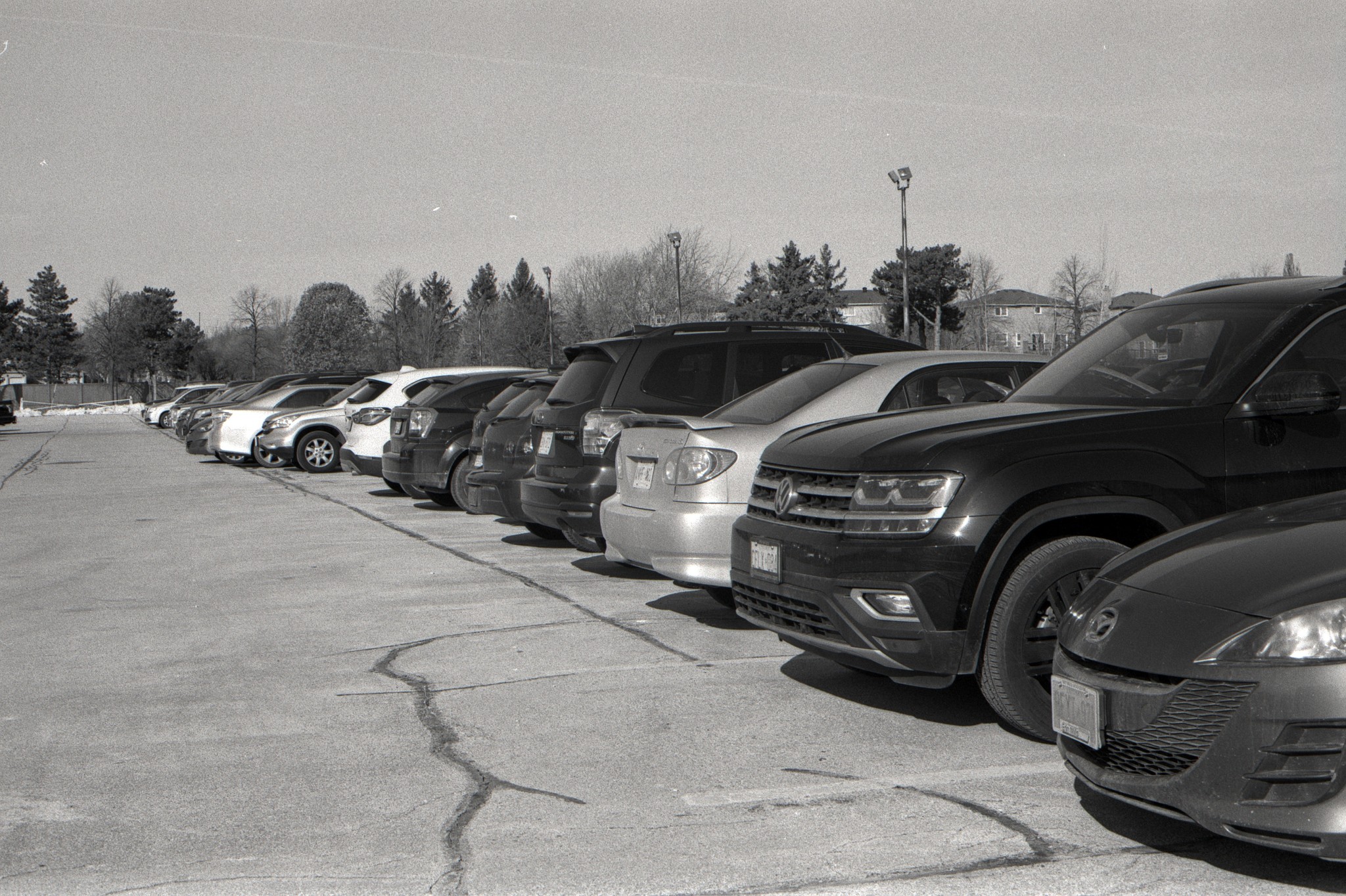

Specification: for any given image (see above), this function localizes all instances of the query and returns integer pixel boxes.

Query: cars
[143,273,1346,866]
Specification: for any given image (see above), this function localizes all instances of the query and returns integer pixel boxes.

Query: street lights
[542,267,554,364]
[666,233,682,322]
[889,168,914,342]
